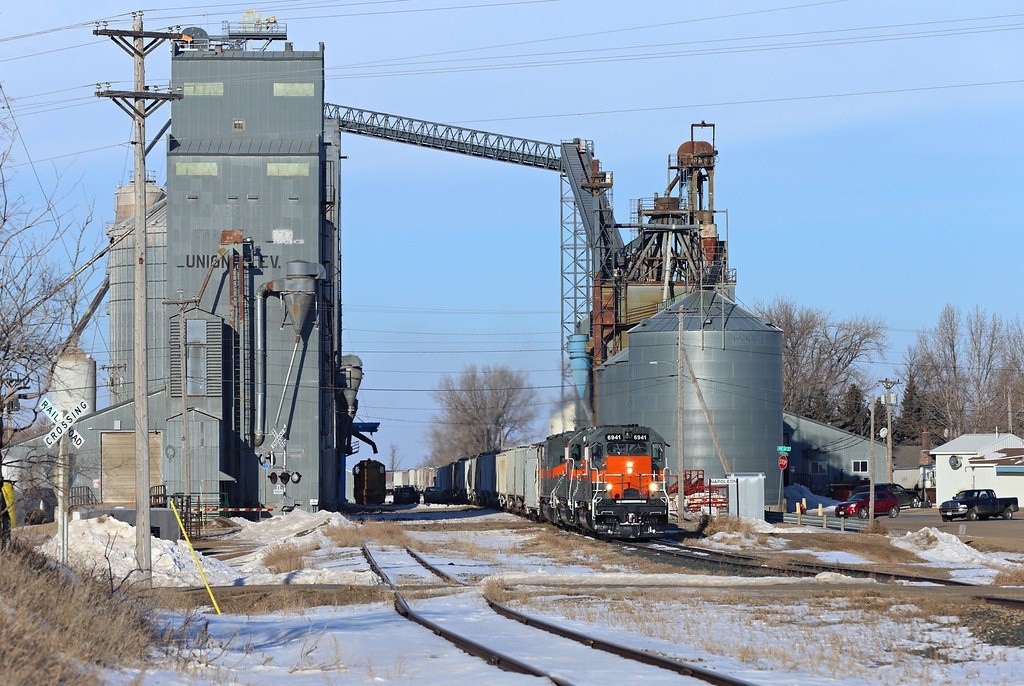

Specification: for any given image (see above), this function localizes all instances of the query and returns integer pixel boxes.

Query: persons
[914,481,918,491]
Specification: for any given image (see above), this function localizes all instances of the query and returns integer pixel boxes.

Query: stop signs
[777,456,788,470]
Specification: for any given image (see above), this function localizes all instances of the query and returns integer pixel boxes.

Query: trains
[386,425,669,541]
[353,459,387,505]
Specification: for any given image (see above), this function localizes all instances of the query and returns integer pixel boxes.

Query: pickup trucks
[938,489,1018,521]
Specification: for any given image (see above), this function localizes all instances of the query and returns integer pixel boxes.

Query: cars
[423,485,451,504]
[393,485,420,505]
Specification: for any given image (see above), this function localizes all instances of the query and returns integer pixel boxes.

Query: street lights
[649,360,684,529]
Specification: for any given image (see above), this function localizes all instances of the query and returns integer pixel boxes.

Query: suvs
[853,484,921,509]
[835,491,901,520]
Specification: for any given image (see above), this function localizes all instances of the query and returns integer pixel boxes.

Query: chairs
[981,494,986,497]
